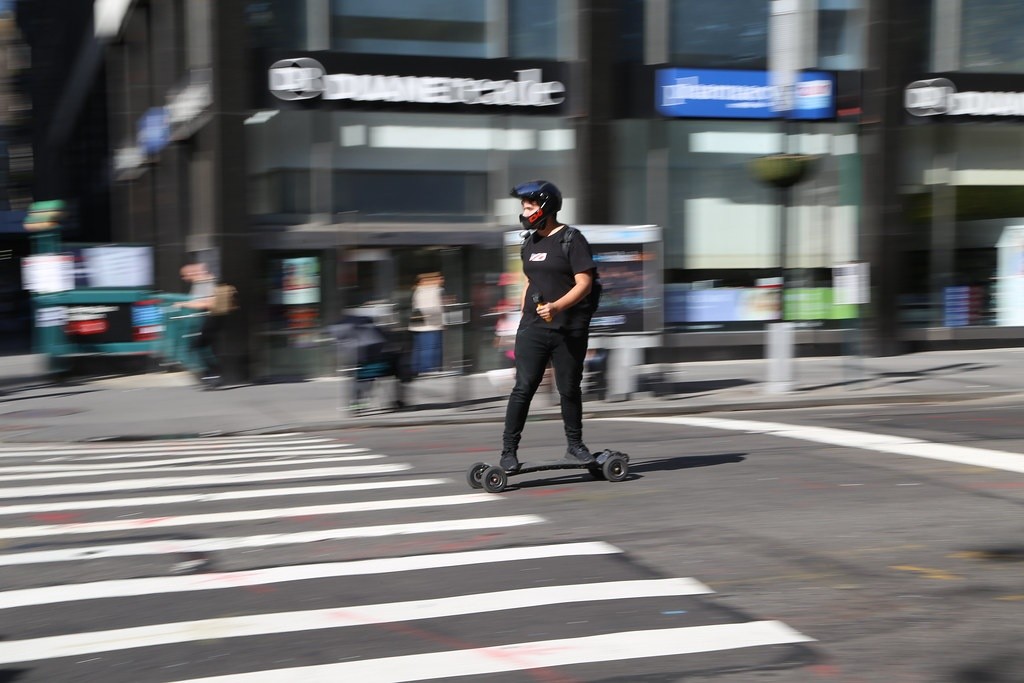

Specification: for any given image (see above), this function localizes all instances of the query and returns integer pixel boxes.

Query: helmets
[509,179,562,230]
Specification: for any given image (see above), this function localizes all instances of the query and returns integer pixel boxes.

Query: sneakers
[499,452,520,471]
[564,444,594,464]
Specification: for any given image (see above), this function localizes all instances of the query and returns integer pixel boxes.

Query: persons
[498,180,603,470]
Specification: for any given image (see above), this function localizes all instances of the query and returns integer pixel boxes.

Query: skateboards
[466,448,630,492]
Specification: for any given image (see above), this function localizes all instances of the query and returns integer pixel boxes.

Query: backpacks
[565,230,603,311]
